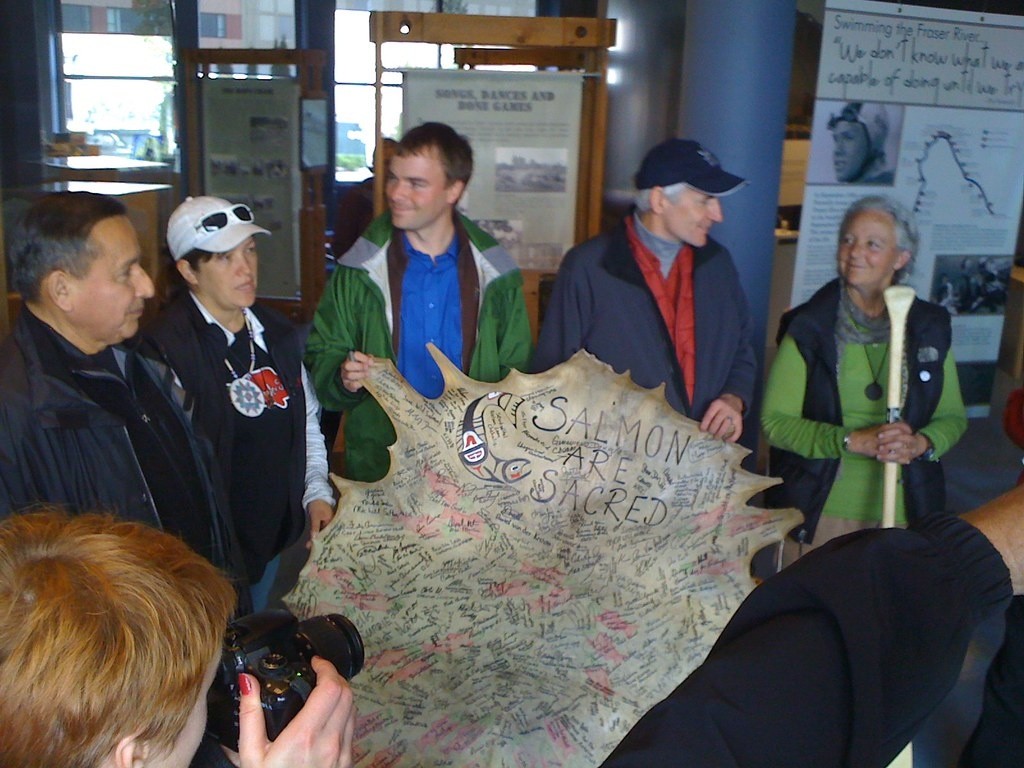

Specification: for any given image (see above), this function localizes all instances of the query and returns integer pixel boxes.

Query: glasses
[827,108,871,146]
[178,203,253,257]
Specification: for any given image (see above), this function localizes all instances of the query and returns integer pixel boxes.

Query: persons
[588,487,1024,768]
[956,388,1024,768]
[0,513,356,768]
[532,136,756,445]
[0,190,253,615]
[332,139,399,262]
[305,122,533,482]
[142,195,337,612]
[826,101,896,186]
[760,193,967,565]
[937,257,1005,316]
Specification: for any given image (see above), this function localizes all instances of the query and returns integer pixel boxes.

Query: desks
[1,180,173,314]
[41,155,171,181]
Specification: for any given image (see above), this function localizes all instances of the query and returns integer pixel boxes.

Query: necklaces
[848,310,889,401]
[225,308,274,417]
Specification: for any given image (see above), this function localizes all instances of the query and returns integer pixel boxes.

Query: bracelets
[842,433,850,448]
[916,445,933,461]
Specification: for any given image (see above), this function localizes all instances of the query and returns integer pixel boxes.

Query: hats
[167,196,272,260]
[633,138,750,197]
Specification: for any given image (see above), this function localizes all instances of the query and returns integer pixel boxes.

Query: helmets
[828,100,890,162]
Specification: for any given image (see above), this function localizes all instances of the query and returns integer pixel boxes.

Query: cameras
[202,604,365,754]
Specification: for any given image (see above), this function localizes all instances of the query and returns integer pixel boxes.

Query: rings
[889,449,896,454]
[728,417,732,421]
